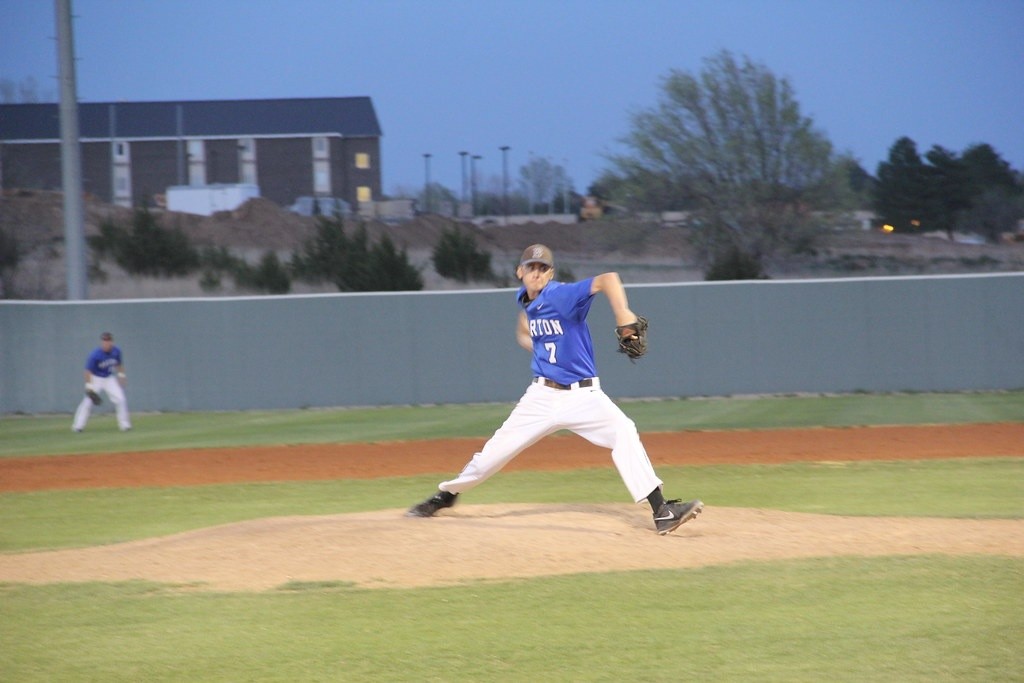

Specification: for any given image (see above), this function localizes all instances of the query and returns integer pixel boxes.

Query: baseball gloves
[86,389,103,406]
[615,316,648,360]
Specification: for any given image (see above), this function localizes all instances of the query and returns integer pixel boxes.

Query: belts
[534,376,592,390]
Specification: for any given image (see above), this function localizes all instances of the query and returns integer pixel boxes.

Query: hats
[100,332,113,341]
[519,244,553,267]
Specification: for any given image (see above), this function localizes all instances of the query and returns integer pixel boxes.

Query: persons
[71,332,132,434]
[408,243,706,538]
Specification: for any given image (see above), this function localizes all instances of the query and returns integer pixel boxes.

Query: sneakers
[653,497,704,535]
[406,492,458,518]
